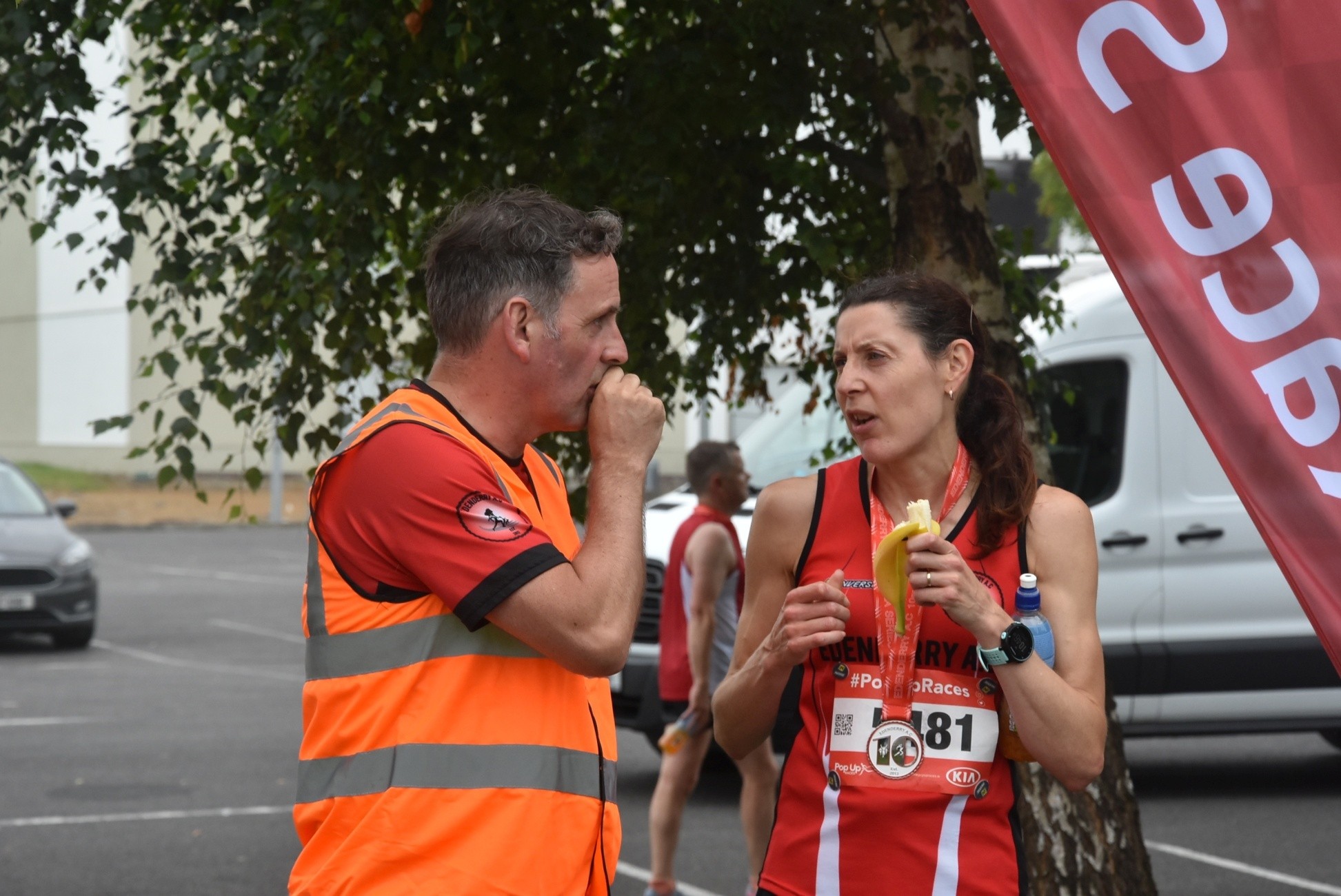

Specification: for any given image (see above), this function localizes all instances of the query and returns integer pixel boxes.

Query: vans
[608,247,1341,779]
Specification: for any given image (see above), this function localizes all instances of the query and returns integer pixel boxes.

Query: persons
[286,185,666,896]
[710,272,1108,896]
[642,440,779,896]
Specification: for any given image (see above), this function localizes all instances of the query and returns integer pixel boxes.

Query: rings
[926,571,933,588]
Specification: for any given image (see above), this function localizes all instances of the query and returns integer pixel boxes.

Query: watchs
[975,621,1035,672]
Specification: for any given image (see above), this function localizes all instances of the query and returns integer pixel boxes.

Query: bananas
[873,499,941,635]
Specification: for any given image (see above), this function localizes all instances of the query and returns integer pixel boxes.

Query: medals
[867,719,924,780]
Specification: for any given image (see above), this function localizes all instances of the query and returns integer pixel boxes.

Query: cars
[0,461,100,653]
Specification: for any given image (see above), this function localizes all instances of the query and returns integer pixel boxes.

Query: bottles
[996,573,1056,763]
[657,710,696,757]
